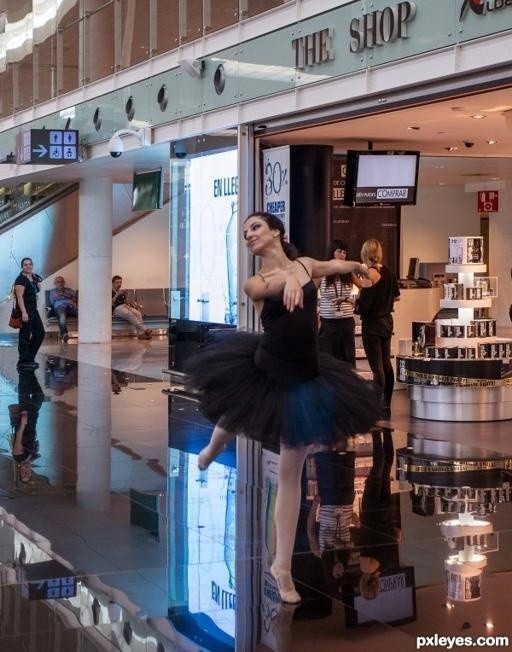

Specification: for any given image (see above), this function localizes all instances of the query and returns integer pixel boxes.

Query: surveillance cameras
[107,124,152,158]
[173,141,187,159]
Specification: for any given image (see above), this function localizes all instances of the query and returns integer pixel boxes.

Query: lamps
[178,60,205,77]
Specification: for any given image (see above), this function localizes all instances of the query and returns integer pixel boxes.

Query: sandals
[198,442,226,471]
[269,563,301,603]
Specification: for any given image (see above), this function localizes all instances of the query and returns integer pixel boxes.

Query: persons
[12,368,45,484]
[49,275,78,342]
[306,421,400,600]
[12,257,45,368]
[313,238,401,420]
[112,275,152,340]
[49,358,74,396]
[111,340,153,395]
[197,211,370,604]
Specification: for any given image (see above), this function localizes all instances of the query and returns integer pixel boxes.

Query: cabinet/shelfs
[396,433,511,568]
[396,264,512,421]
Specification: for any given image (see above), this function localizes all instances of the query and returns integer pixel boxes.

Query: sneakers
[16,366,39,375]
[383,407,391,418]
[16,360,40,367]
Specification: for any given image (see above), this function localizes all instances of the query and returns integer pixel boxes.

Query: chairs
[44,288,169,338]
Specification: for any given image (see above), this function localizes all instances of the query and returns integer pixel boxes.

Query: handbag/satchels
[8,298,23,329]
[8,404,21,434]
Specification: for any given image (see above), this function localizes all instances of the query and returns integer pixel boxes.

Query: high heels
[271,603,301,636]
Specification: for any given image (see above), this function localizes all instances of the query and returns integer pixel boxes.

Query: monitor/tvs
[130,487,161,538]
[408,257,421,281]
[343,150,420,207]
[344,565,417,631]
[132,166,164,211]
